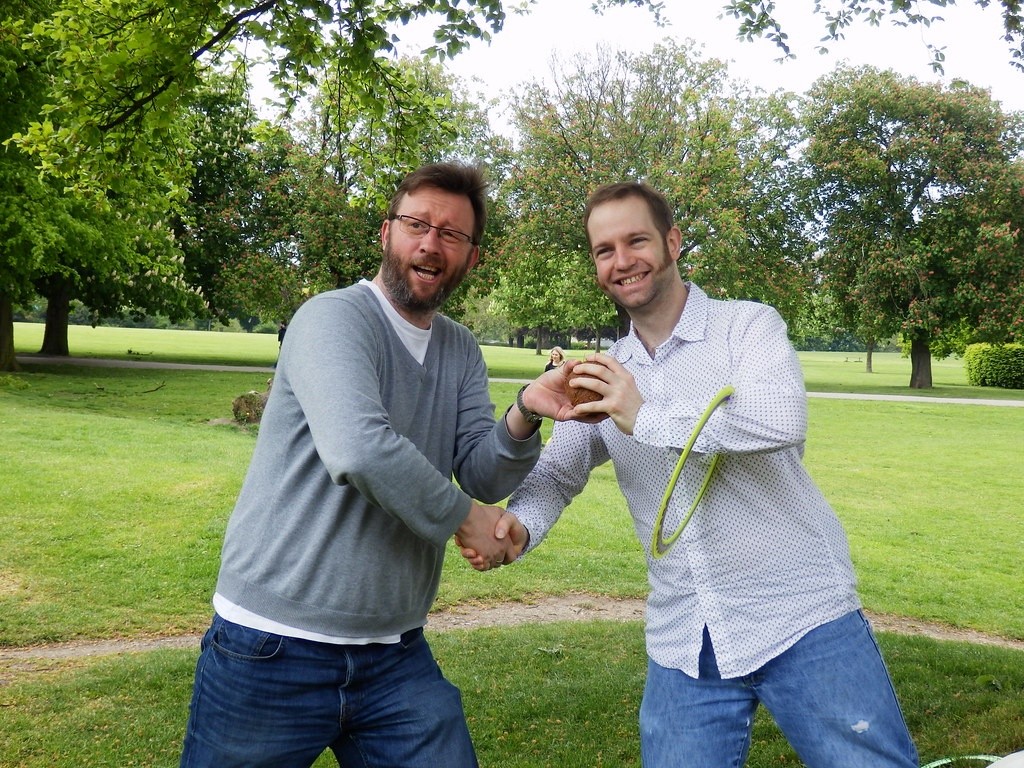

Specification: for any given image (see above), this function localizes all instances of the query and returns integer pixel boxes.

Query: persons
[180,164,588,767]
[455,180,919,767]
[545,346,565,372]
[274,320,288,367]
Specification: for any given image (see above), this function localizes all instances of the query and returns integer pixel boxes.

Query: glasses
[388,215,478,252]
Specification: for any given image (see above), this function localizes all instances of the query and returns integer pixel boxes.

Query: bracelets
[516,384,541,424]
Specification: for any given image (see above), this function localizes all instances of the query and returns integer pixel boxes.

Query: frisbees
[651,383,736,561]
